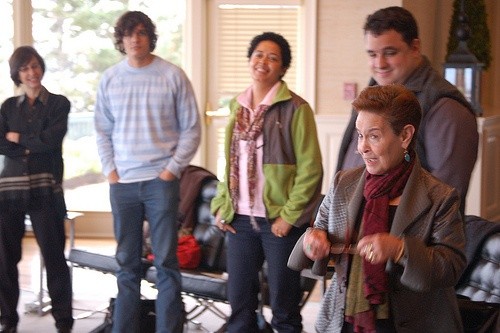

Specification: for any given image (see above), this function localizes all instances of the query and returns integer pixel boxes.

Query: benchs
[451,217,500,333]
[67,173,323,333]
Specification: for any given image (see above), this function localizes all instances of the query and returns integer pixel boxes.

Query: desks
[24,210,86,315]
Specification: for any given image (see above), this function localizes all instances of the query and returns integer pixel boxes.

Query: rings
[305,243,311,252]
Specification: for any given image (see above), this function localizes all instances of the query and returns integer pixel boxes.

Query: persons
[0,45,74,333]
[335,6,479,216]
[93,11,202,333]
[210,31,324,333]
[286,84,470,333]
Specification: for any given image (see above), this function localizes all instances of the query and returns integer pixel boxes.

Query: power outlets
[343,82,357,101]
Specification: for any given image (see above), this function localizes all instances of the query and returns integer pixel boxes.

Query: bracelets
[393,241,405,263]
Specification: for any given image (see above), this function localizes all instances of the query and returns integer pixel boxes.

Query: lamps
[439,0,491,69]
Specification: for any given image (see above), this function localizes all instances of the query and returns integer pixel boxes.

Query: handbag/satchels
[104,297,187,333]
[148,236,202,268]
